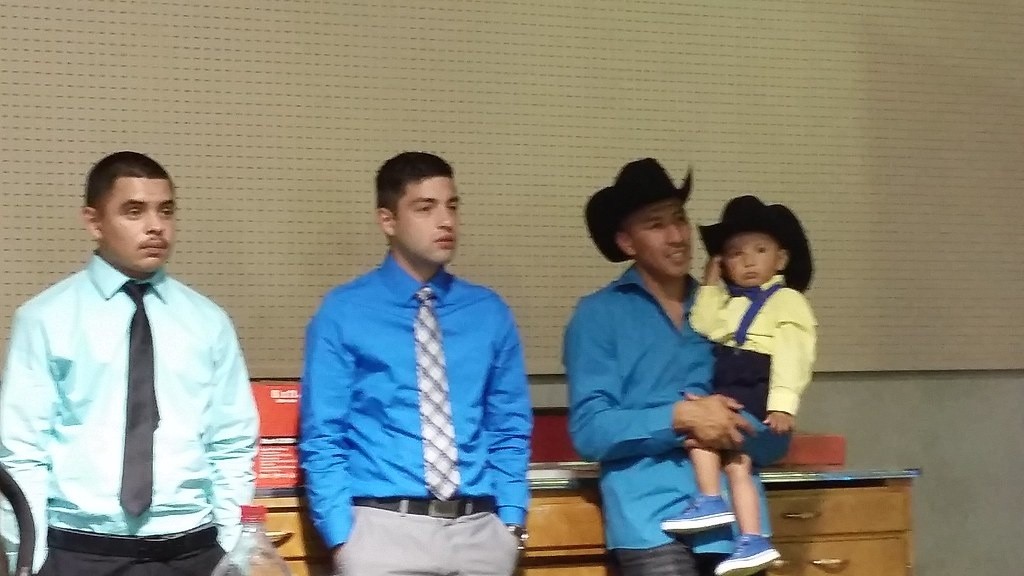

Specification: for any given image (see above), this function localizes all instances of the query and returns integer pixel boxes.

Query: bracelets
[507,525,531,542]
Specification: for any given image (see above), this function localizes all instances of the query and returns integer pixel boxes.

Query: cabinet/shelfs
[230,464,929,576]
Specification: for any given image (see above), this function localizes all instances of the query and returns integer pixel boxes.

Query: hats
[586,158,693,263]
[699,195,811,294]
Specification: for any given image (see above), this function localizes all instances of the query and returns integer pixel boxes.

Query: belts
[49,526,218,560]
[351,496,496,518]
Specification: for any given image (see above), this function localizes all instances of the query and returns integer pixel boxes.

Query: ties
[120,283,160,518]
[414,287,462,502]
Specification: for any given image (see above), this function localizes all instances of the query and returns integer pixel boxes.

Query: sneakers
[714,534,781,576]
[661,493,736,534]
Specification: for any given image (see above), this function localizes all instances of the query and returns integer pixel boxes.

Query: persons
[660,194,819,576]
[297,151,534,576]
[0,150,262,576]
[563,156,794,576]
